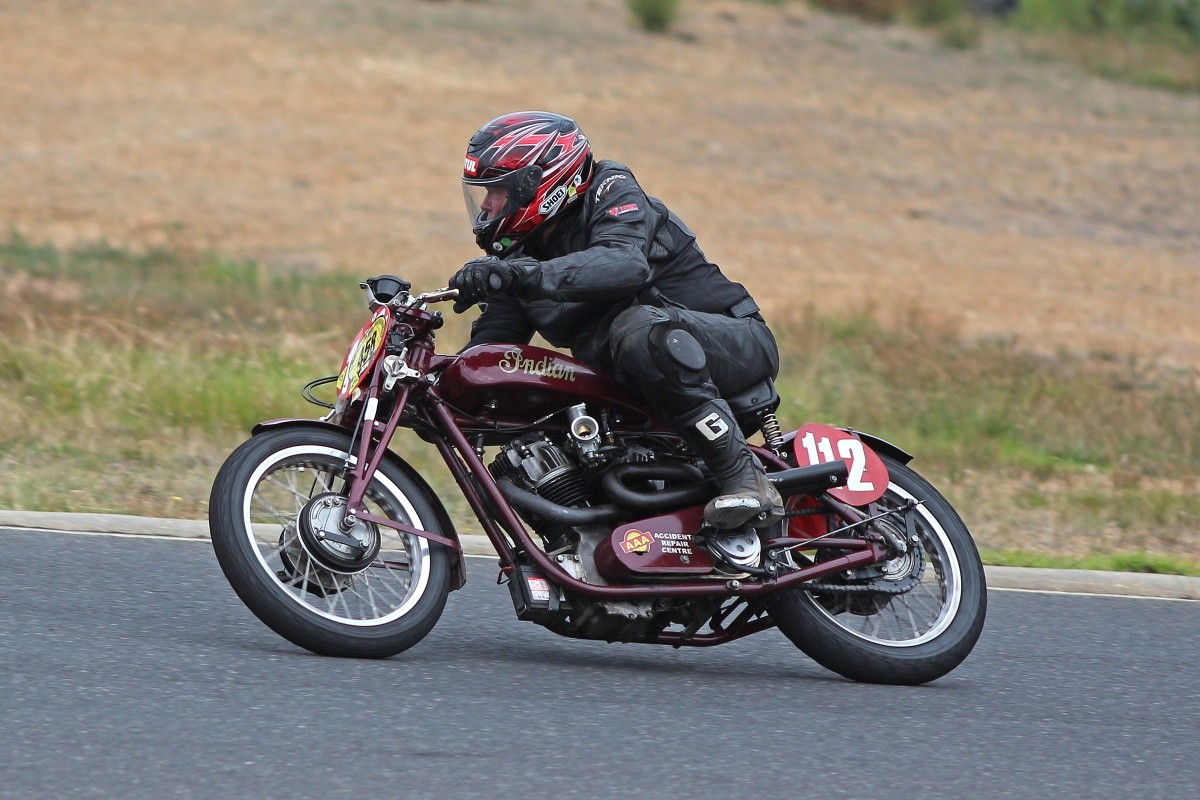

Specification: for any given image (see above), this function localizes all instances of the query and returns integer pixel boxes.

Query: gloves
[448,248,543,314]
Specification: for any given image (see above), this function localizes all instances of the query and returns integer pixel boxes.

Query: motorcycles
[208,272,987,686]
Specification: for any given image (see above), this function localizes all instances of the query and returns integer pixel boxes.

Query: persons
[449,111,786,534]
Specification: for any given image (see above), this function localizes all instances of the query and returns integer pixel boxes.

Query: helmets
[460,111,596,258]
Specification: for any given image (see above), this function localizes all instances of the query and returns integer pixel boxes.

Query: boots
[671,397,785,528]
